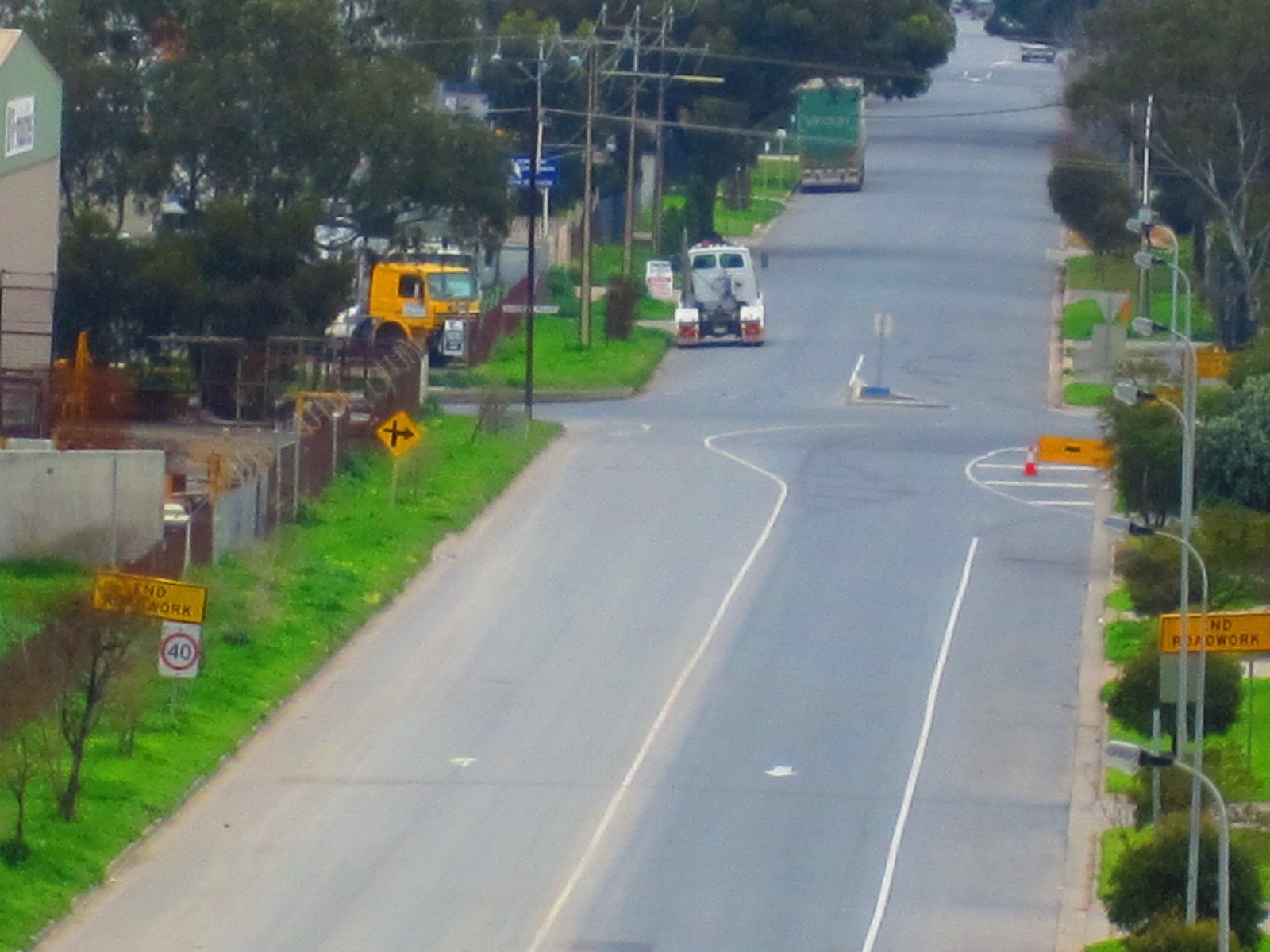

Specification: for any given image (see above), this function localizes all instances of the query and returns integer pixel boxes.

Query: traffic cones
[1024,447,1037,477]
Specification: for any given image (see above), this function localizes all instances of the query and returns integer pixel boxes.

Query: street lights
[490,54,580,426]
[1101,515,1209,931]
[1110,378,1194,764]
[1100,738,1230,952]
[1110,216,1198,526]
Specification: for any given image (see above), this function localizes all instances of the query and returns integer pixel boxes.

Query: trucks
[797,75,865,194]
[324,237,483,367]
[674,239,769,350]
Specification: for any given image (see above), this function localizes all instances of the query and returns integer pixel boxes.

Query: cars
[1020,41,1056,63]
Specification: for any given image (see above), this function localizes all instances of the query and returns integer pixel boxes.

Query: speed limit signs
[158,621,202,680]
